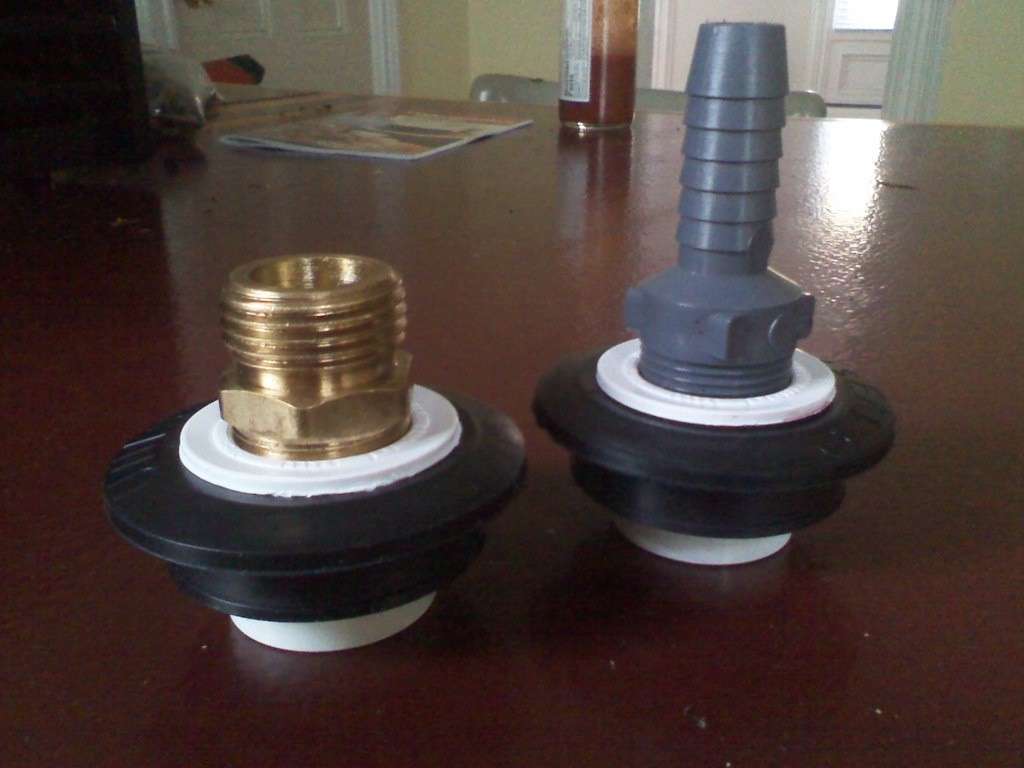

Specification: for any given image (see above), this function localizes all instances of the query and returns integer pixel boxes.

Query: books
[220,108,534,160]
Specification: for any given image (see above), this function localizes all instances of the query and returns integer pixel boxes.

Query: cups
[559,0,639,129]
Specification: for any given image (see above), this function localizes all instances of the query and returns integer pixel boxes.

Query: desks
[0,80,1024,768]
